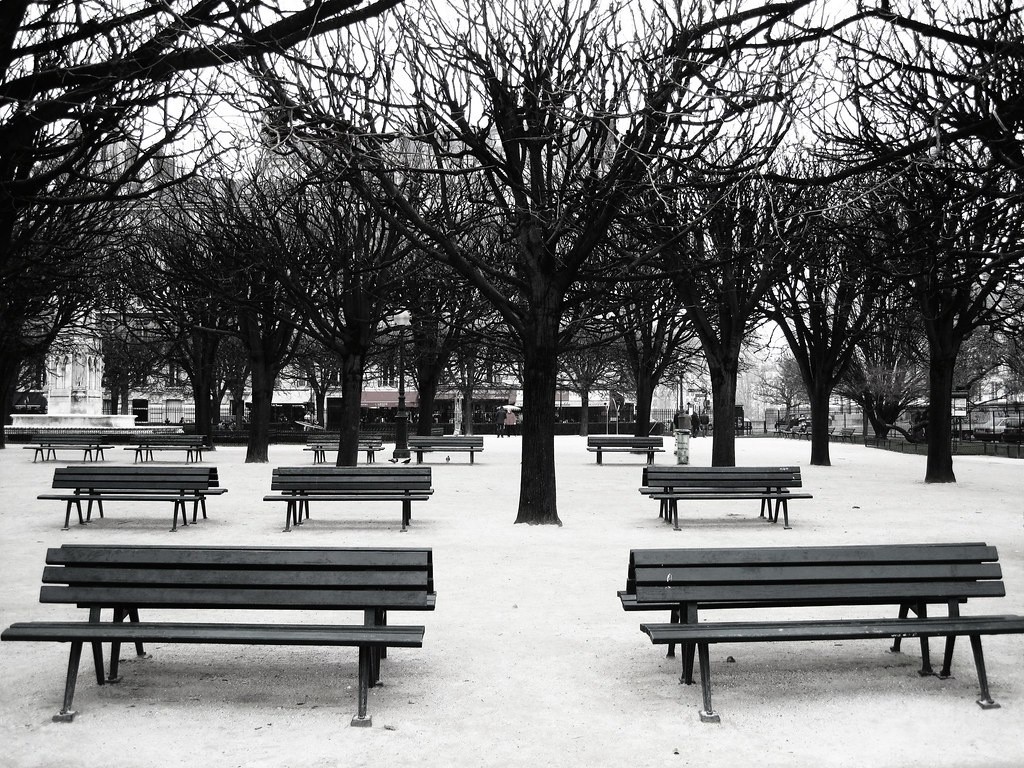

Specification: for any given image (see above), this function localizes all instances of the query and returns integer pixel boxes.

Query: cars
[974,417,1024,441]
[774,413,833,430]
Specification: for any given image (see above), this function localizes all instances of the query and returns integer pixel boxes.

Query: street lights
[390,308,412,464]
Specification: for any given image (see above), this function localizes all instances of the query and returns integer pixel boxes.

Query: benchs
[639,465,813,531]
[37,466,228,532]
[124,435,213,465]
[1,545,437,728]
[405,436,483,465]
[772,425,856,445]
[23,434,114,464]
[304,434,386,465]
[263,466,434,532]
[586,437,665,466]
[617,542,1024,723]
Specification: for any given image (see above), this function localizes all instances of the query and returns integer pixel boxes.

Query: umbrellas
[496,405,521,412]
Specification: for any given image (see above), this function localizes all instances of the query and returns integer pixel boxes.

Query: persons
[278,414,288,424]
[504,409,517,437]
[684,410,692,429]
[673,410,680,434]
[241,417,250,423]
[691,412,699,438]
[699,410,709,437]
[216,418,236,431]
[179,417,185,424]
[164,418,170,426]
[494,407,507,438]
